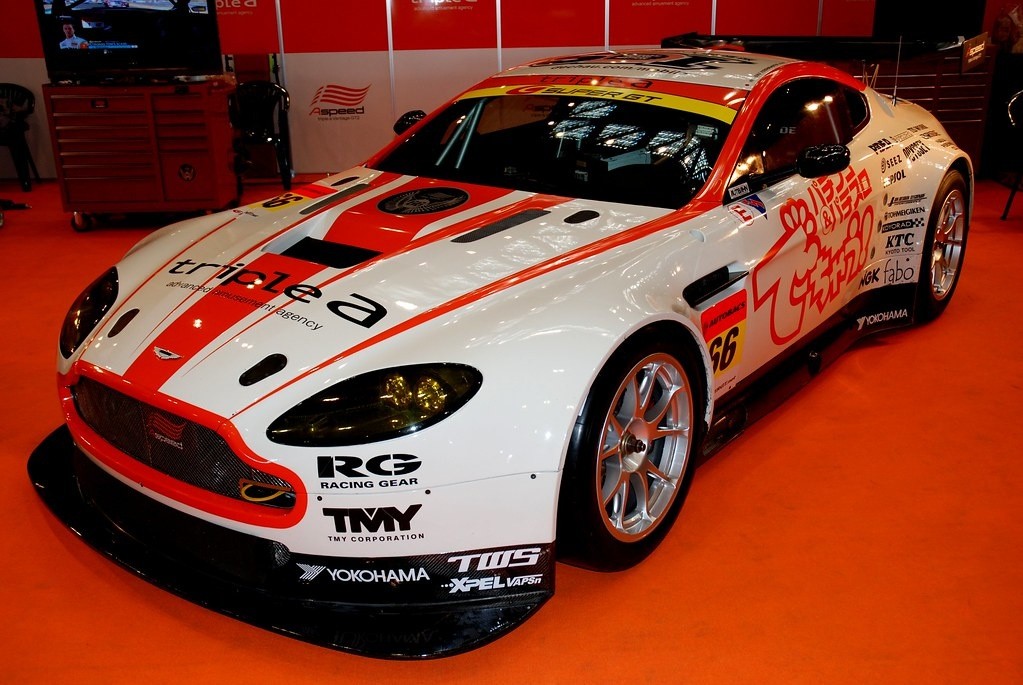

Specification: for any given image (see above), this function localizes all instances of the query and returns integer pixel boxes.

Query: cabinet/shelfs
[853,32,1000,176]
[42,78,241,232]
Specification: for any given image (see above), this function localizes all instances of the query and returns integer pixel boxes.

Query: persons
[59,23,88,48]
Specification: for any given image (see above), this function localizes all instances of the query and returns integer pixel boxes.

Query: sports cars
[27,48,974,661]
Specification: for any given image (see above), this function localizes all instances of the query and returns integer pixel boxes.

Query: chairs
[0,82,41,192]
[228,79,292,196]
[1001,89,1023,221]
